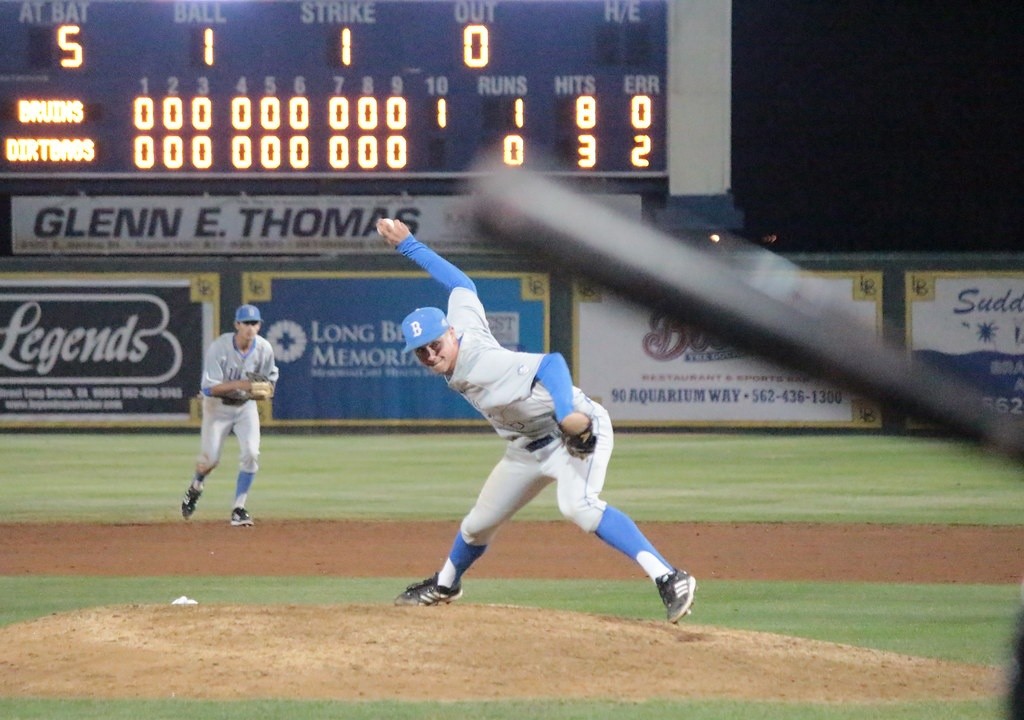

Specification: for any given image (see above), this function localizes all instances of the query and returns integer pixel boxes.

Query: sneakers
[231,507,253,526]
[394,573,463,607]
[656,567,697,624]
[181,481,203,518]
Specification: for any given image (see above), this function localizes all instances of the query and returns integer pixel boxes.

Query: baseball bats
[459,134,1024,464]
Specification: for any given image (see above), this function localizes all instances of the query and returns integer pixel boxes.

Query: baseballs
[376,217,396,237]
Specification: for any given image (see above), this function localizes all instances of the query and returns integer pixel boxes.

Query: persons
[375,216,697,624]
[182,305,278,528]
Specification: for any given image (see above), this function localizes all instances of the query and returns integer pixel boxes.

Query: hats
[401,307,450,355]
[236,304,260,322]
